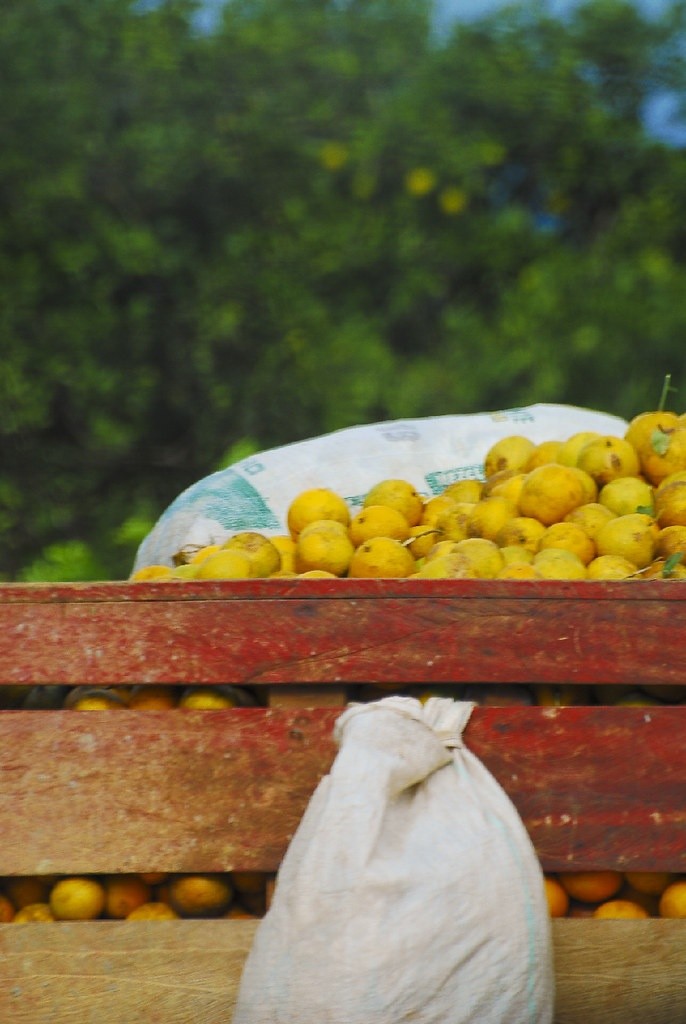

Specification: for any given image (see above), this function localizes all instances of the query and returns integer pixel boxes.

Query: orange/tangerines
[0,410,686,924]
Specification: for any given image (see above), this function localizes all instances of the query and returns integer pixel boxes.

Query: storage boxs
[0,577,686,1024]
